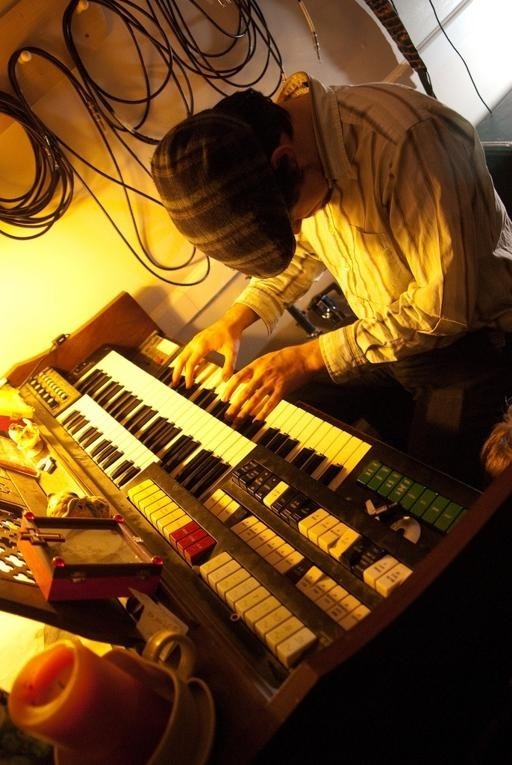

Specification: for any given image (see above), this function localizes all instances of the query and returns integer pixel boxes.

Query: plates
[177,676,217,764]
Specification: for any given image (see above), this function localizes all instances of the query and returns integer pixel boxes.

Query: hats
[149,107,298,280]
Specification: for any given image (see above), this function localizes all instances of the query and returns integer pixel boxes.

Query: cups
[55,631,196,764]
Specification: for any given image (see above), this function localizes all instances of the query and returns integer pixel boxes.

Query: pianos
[4,287,509,741]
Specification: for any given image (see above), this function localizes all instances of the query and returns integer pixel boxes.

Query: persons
[142,70,512,425]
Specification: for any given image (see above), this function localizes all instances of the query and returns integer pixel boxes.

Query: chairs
[475,141,512,217]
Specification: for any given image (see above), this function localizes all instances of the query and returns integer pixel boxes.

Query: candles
[7,633,167,759]
